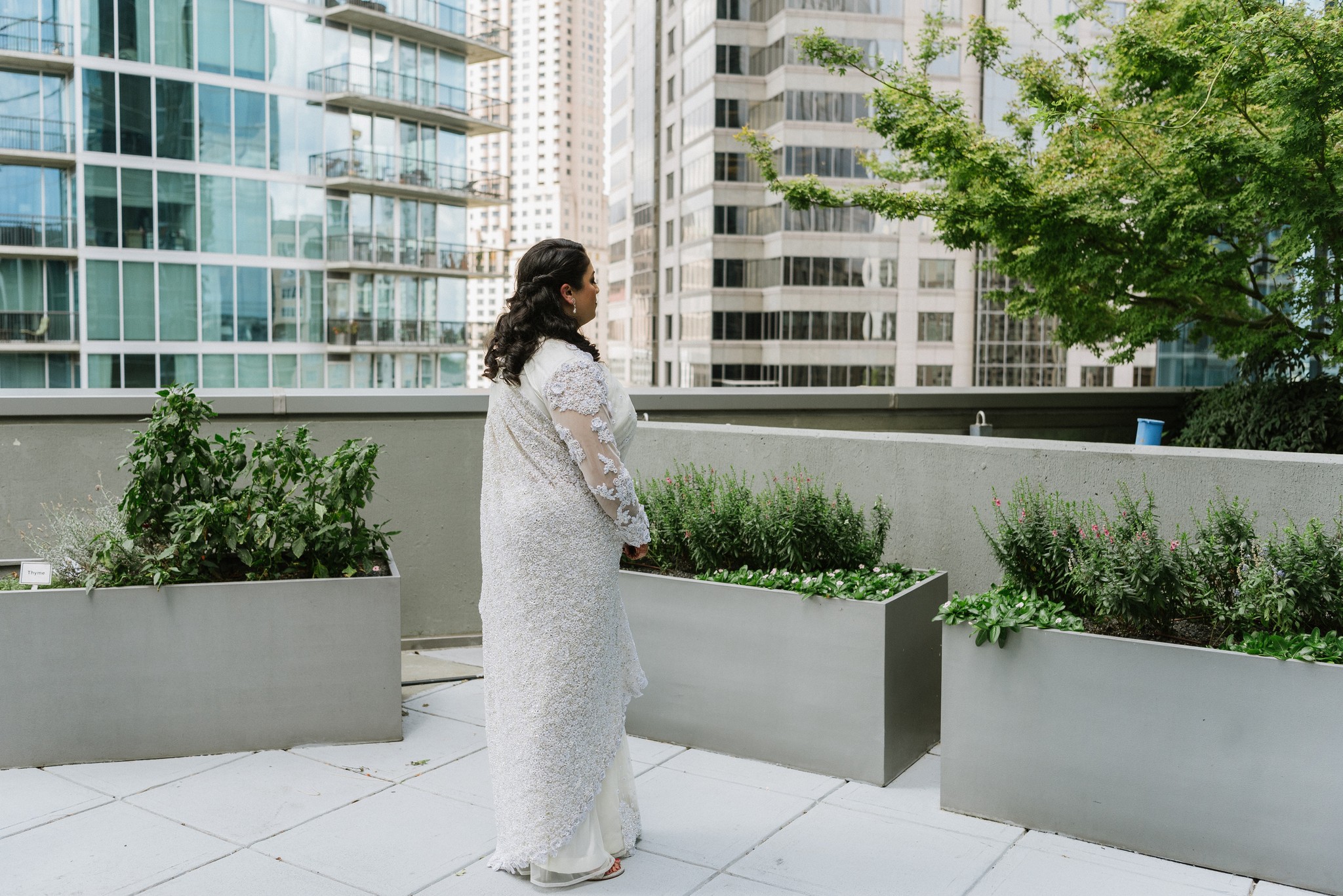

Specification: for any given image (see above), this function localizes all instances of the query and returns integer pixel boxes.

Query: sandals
[577,857,625,881]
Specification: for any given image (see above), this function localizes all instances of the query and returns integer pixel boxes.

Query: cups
[1134,417,1164,446]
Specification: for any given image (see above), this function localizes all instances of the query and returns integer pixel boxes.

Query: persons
[478,238,651,882]
